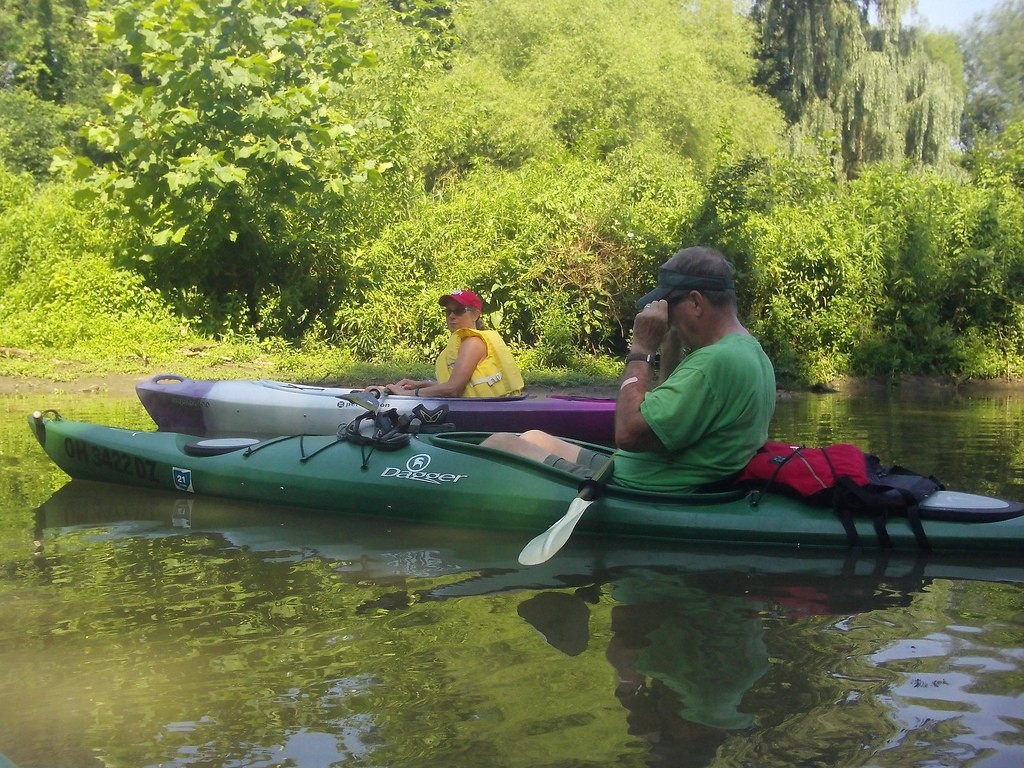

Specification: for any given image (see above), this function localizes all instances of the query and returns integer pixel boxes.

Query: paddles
[517,452,614,568]
[334,387,391,413]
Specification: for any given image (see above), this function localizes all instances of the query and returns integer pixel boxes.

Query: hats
[438,289,482,312]
[636,265,729,308]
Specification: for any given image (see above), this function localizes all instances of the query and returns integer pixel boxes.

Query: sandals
[385,403,456,434]
[345,410,411,452]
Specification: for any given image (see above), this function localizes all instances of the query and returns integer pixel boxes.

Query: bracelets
[624,353,655,368]
[415,388,420,397]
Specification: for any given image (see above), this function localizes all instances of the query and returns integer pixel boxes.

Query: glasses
[442,308,478,317]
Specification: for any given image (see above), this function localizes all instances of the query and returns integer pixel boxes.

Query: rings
[644,305,651,308]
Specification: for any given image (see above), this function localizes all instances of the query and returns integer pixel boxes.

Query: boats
[29,470,1024,594]
[25,407,1024,553]
[134,373,617,452]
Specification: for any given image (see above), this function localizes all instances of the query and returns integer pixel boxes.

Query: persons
[478,248,776,495]
[350,290,524,398]
